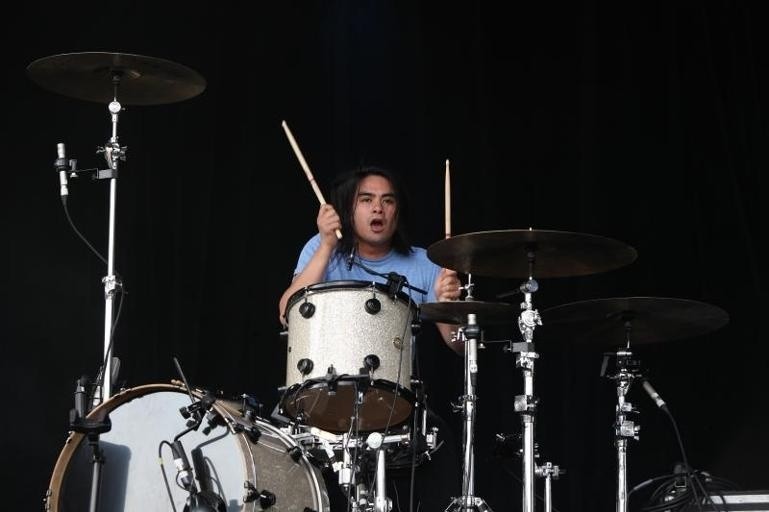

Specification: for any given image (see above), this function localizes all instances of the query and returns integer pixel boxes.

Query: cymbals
[543,297,729,347]
[418,302,517,326]
[427,230,640,280]
[29,52,205,107]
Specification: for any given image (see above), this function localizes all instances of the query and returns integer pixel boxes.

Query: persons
[279,166,466,512]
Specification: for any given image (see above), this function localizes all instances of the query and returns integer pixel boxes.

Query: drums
[46,384,332,510]
[282,280,415,433]
